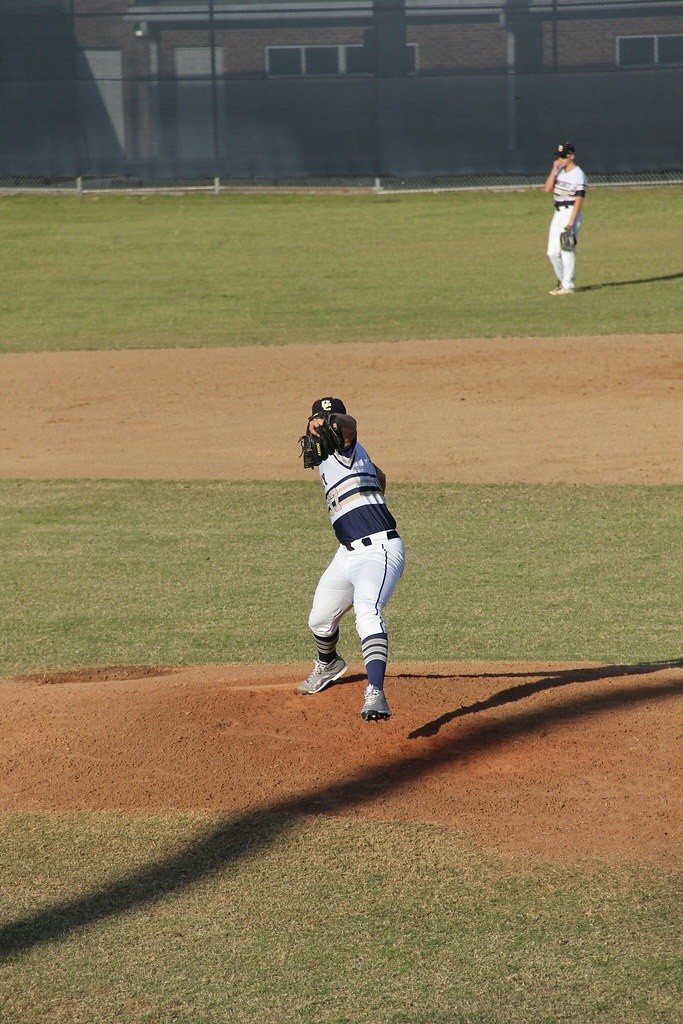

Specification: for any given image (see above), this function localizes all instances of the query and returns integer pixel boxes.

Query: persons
[543,142,588,296]
[296,398,405,722]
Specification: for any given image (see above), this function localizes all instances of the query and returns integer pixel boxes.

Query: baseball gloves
[559,224,578,252]
[296,411,358,471]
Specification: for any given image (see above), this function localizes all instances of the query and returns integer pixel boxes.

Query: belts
[555,205,568,210]
[346,531,399,551]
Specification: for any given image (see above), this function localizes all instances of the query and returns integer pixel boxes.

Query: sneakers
[549,282,575,296]
[360,683,391,723]
[295,656,347,694]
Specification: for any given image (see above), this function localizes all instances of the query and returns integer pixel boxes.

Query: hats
[555,142,574,158]
[308,397,346,418]
